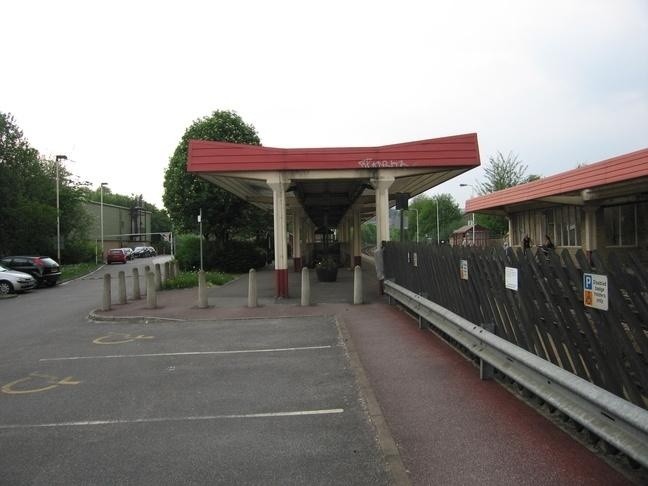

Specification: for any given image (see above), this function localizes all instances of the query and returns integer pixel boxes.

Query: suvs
[0,255,62,289]
[106,249,127,265]
[132,245,151,258]
[148,246,157,257]
[121,247,135,261]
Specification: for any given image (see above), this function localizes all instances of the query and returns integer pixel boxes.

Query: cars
[0,264,37,298]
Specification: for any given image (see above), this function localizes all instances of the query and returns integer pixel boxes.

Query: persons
[541,235,554,257]
[522,234,531,256]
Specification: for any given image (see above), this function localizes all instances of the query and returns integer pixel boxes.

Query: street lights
[459,183,475,246]
[426,197,440,247]
[55,154,69,269]
[100,182,108,263]
[408,207,420,244]
